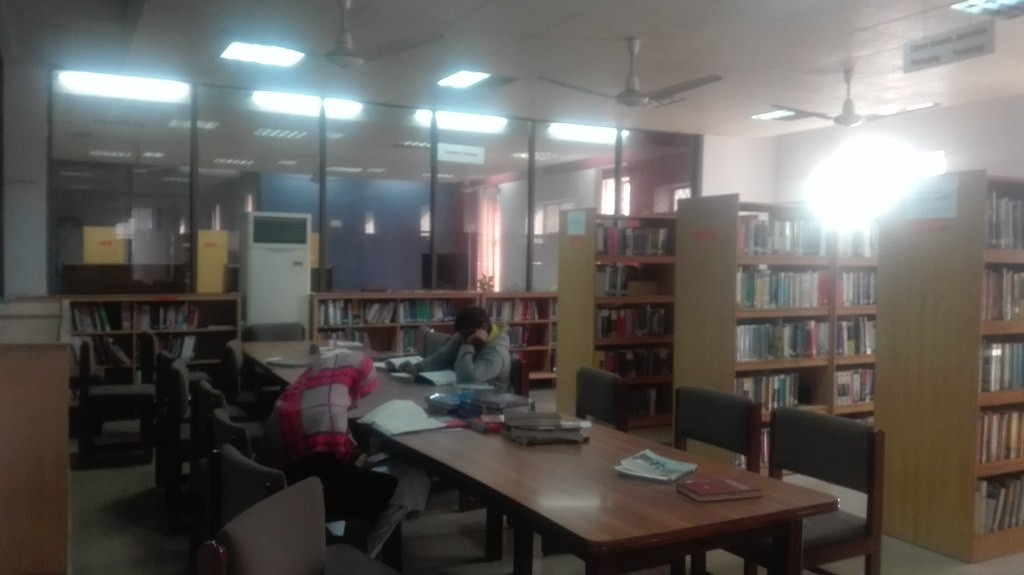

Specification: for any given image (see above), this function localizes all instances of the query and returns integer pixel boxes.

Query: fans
[772,68,942,127]
[272,0,446,85]
[537,37,724,108]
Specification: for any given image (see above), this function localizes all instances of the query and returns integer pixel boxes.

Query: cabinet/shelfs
[311,291,481,358]
[868,167,1024,564]
[674,192,877,477]
[558,207,675,430]
[481,292,557,391]
[57,291,242,437]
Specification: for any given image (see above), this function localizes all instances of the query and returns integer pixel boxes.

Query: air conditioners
[239,209,314,335]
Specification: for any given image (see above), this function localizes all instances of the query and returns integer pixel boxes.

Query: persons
[397,306,512,396]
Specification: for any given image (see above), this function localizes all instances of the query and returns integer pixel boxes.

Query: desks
[243,337,841,575]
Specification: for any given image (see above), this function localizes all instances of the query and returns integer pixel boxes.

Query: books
[73,299,200,365]
[317,297,558,373]
[737,216,878,471]
[676,477,764,503]
[423,391,536,435]
[594,223,673,380]
[977,191,1024,536]
[613,448,698,484]
[372,352,495,393]
[502,408,593,448]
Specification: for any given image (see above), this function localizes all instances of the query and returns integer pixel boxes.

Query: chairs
[222,338,280,422]
[458,356,530,514]
[506,366,629,558]
[190,378,229,564]
[220,444,399,575]
[242,321,307,407]
[670,385,762,575]
[194,476,327,575]
[689,407,885,575]
[81,338,156,465]
[416,324,432,357]
[211,408,400,571]
[139,326,212,442]
[155,349,267,538]
[425,330,452,356]
